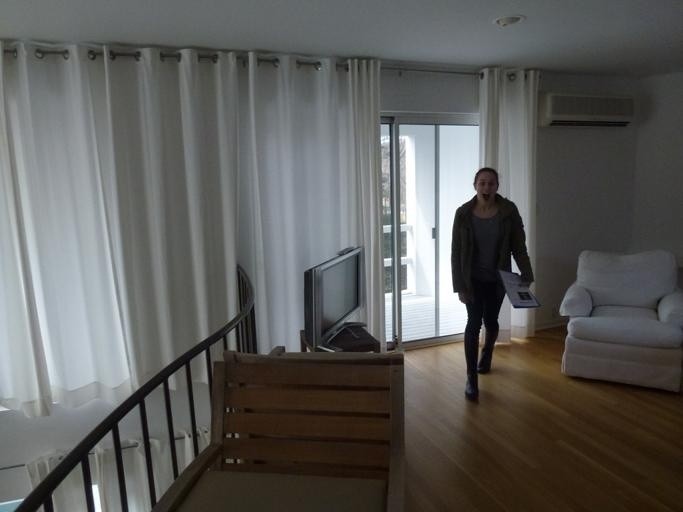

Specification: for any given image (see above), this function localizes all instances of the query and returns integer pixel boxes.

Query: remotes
[338,246,355,254]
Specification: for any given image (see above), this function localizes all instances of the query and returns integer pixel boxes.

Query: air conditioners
[538,92,635,128]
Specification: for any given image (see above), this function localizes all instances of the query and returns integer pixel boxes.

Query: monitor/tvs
[304,247,367,352]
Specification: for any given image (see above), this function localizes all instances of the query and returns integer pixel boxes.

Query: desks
[301,329,380,353]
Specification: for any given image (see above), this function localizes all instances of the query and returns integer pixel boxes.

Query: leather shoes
[465,381,478,400]
[478,358,491,374]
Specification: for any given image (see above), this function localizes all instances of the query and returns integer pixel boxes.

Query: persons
[452,167,534,399]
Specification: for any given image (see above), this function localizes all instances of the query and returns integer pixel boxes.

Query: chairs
[558,249,683,392]
[146,346,407,512]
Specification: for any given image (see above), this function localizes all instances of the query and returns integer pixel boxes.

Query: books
[499,270,539,308]
